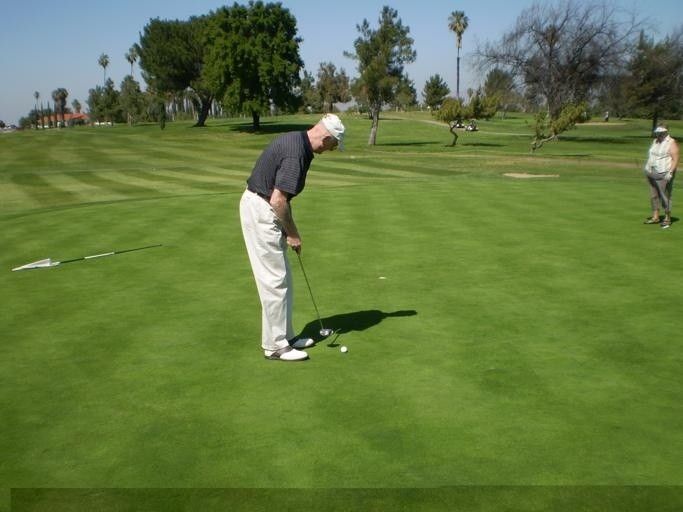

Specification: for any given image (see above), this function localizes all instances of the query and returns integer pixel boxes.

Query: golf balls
[341,345,346,353]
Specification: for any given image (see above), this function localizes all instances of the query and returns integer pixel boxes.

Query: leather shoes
[264,346,308,361]
[288,337,314,348]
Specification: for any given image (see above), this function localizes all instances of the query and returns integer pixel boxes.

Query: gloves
[664,172,673,183]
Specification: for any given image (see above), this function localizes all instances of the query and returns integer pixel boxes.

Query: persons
[603,111,609,123]
[642,123,679,230]
[237,111,347,363]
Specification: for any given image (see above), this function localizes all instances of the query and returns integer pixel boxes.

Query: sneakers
[662,220,671,226]
[644,218,660,224]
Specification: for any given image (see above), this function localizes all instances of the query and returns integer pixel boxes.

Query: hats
[322,113,344,153]
[654,128,667,132]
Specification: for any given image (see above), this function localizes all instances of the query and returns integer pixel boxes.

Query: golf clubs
[294,249,334,337]
